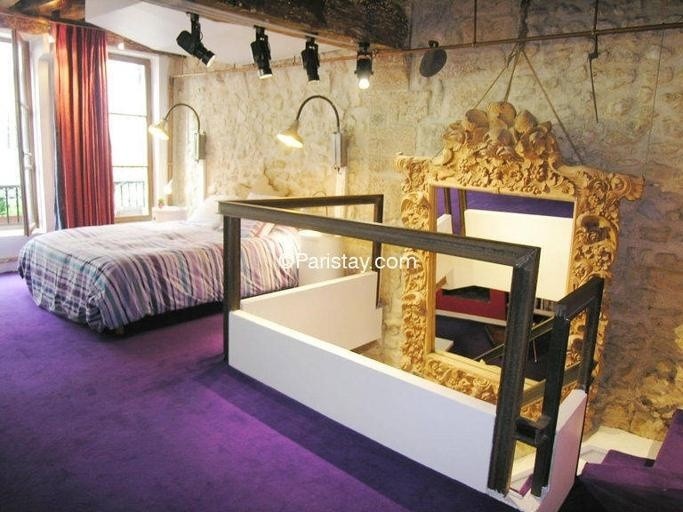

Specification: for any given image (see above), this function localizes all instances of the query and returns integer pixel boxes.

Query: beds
[15,197,358,337]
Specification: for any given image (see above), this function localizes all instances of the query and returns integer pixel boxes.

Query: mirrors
[392,102,645,432]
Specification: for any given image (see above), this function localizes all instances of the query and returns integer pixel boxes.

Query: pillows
[191,198,275,238]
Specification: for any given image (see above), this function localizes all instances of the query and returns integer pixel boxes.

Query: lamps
[277,96,347,170]
[148,103,206,162]
[176,10,373,91]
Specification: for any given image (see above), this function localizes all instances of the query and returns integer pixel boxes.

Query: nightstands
[297,230,342,286]
[152,206,187,222]
